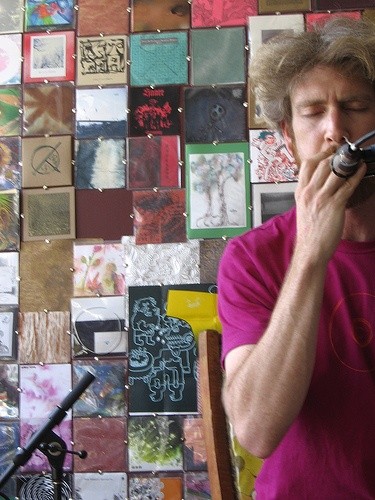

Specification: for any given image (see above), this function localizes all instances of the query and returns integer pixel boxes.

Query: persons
[218,15,375,500]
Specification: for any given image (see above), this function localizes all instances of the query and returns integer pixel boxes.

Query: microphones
[329,142,360,179]
